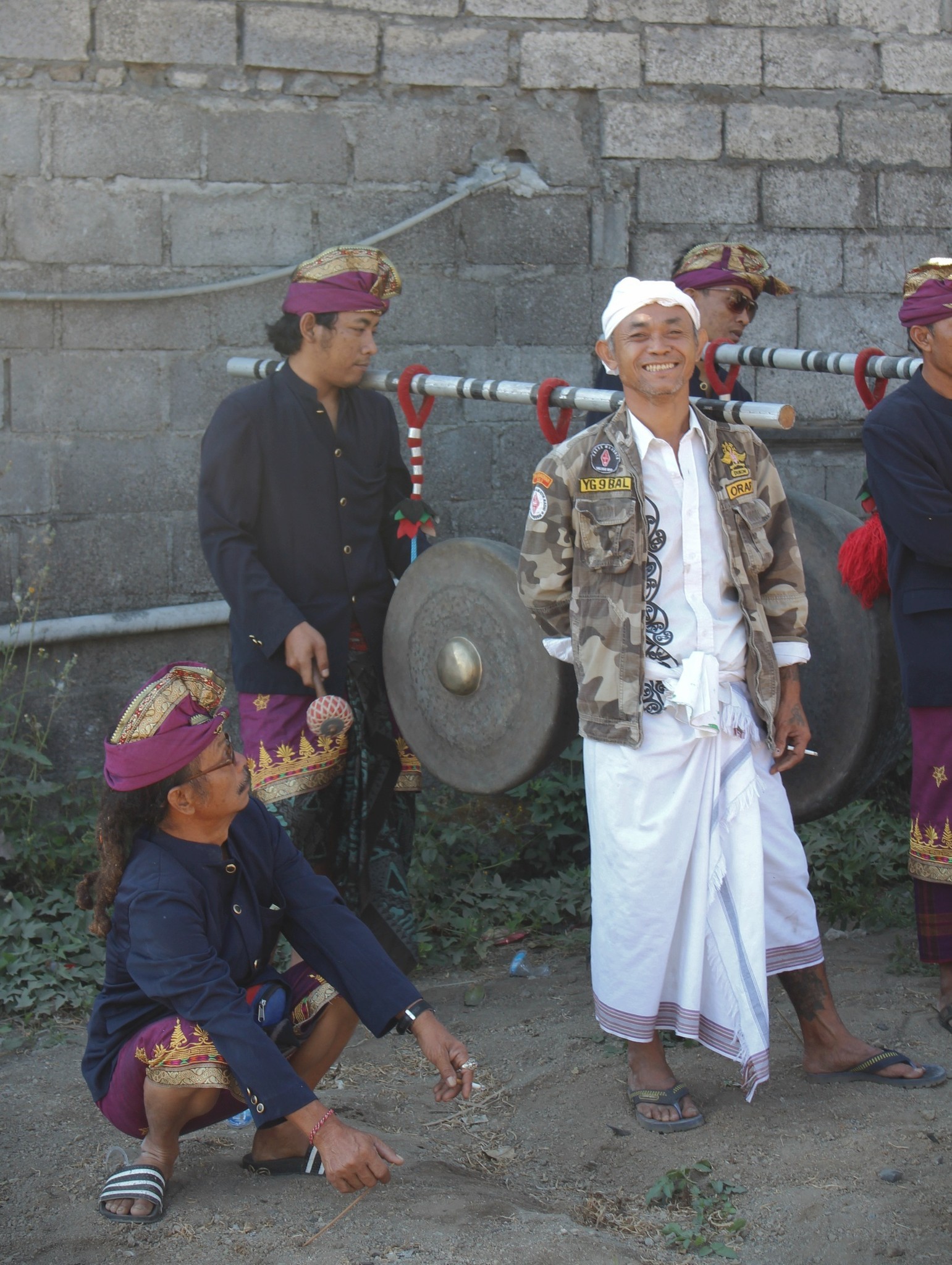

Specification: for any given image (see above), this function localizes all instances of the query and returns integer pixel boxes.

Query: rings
[455,1069,461,1072]
[461,1058,479,1071]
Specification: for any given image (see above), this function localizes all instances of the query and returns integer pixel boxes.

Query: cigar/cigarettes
[456,1079,482,1090]
[786,745,818,756]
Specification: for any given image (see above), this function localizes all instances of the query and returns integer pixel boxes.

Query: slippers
[937,1005,952,1032]
[98,1164,167,1224]
[627,1083,706,1134]
[807,1047,947,1089]
[243,1145,326,1175]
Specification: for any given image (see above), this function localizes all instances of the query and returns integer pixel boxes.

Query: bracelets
[308,1108,334,1146]
[396,999,437,1035]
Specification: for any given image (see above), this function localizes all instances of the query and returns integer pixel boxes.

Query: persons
[585,240,793,1046]
[515,277,946,1132]
[81,662,476,1224]
[860,256,951,1034]
[194,246,431,981]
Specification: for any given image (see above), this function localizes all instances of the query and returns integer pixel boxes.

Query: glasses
[176,733,236,786]
[705,286,759,325]
